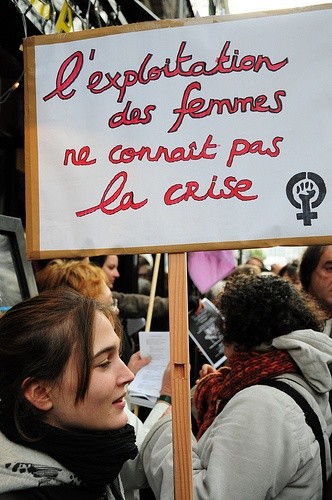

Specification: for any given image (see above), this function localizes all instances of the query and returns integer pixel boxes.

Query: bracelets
[158,395,171,404]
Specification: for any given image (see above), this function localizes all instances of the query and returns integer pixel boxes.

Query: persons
[0,287,138,500]
[140,273,331,500]
[33,254,301,500]
[300,244,332,340]
